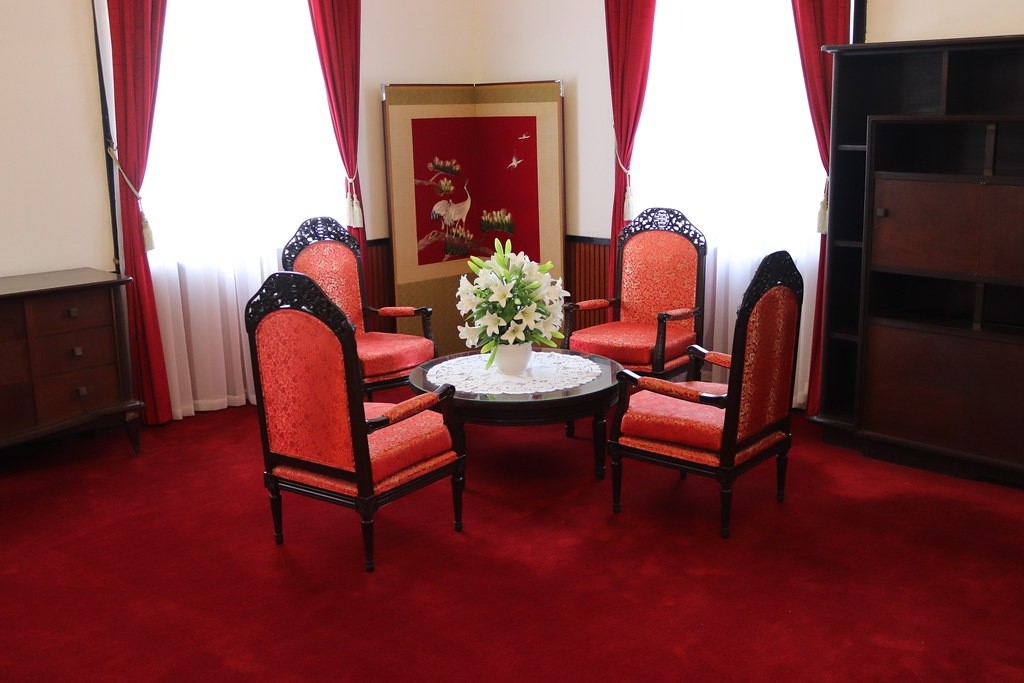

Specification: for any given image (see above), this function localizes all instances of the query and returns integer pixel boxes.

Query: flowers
[455,238,572,371]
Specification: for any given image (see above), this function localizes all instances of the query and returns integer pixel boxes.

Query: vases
[496,343,531,378]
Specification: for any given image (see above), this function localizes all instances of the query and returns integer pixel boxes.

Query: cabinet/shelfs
[806,33,1024,492]
[0,267,145,455]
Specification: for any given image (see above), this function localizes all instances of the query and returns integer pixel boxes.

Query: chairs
[606,251,804,538]
[281,216,439,402]
[561,208,708,439]
[244,270,467,573]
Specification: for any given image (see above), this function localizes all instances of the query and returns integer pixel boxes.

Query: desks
[407,349,625,532]
